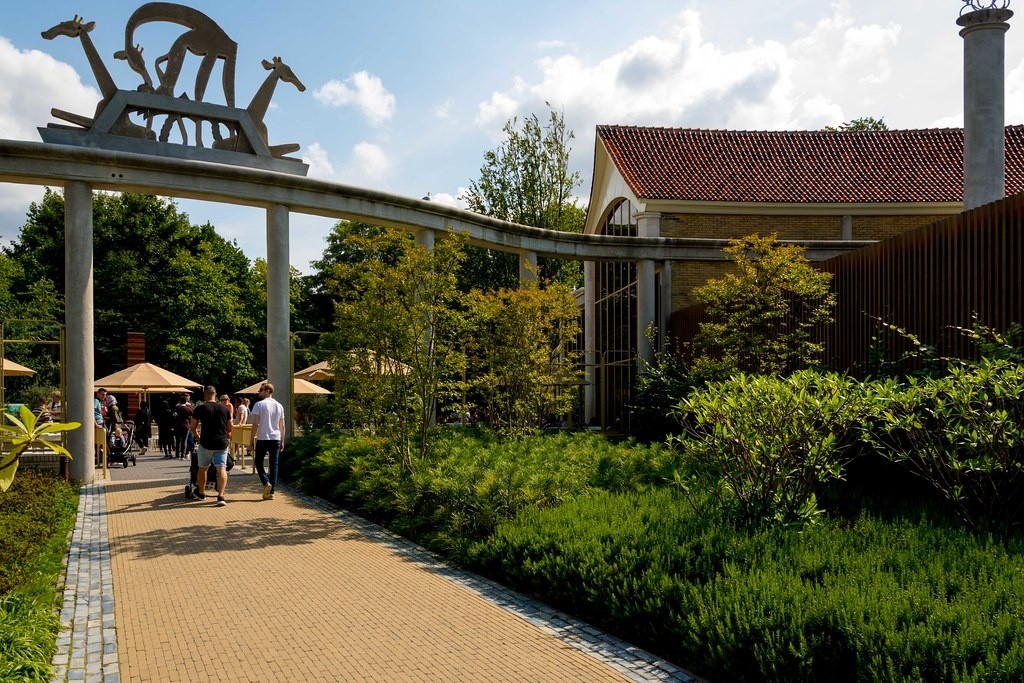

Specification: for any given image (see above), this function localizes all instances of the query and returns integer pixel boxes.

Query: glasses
[221,398,228,402]
[260,388,265,391]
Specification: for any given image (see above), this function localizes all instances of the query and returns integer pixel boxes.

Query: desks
[231,424,271,475]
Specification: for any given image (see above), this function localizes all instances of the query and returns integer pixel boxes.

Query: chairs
[149,426,159,451]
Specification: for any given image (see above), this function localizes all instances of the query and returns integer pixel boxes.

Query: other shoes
[268,493,274,499]
[262,482,272,500]
[139,446,148,455]
[193,486,206,501]
[177,453,190,460]
[163,454,173,459]
[217,495,226,506]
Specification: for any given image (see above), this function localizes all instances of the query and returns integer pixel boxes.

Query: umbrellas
[94,363,204,402]
[293,348,415,380]
[235,378,333,394]
[0,357,37,378]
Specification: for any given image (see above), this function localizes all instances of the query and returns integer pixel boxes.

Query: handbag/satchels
[226,453,235,471]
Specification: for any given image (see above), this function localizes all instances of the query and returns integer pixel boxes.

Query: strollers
[106,420,136,468]
[184,439,222,499]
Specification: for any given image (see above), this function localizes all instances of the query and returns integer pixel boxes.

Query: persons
[48,391,62,421]
[94,389,130,464]
[132,401,149,455]
[220,395,234,438]
[191,386,233,506]
[233,398,253,456]
[32,397,53,439]
[159,394,200,460]
[250,383,285,500]
